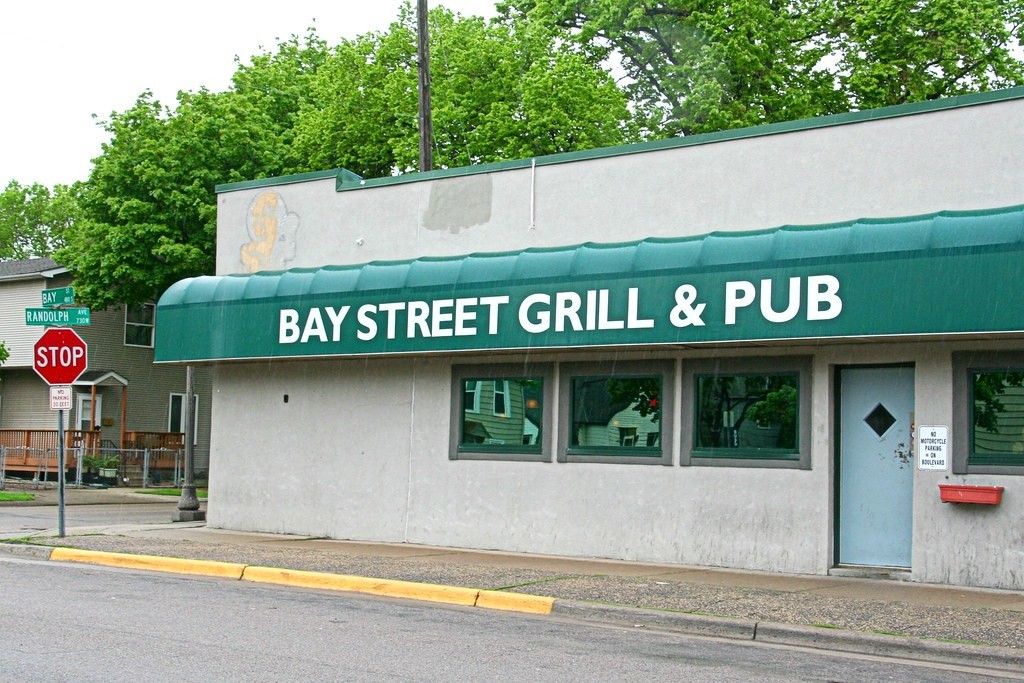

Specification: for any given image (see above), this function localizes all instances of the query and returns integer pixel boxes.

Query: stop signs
[33,328,89,387]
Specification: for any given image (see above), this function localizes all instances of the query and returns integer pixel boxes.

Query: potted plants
[83,454,120,485]
